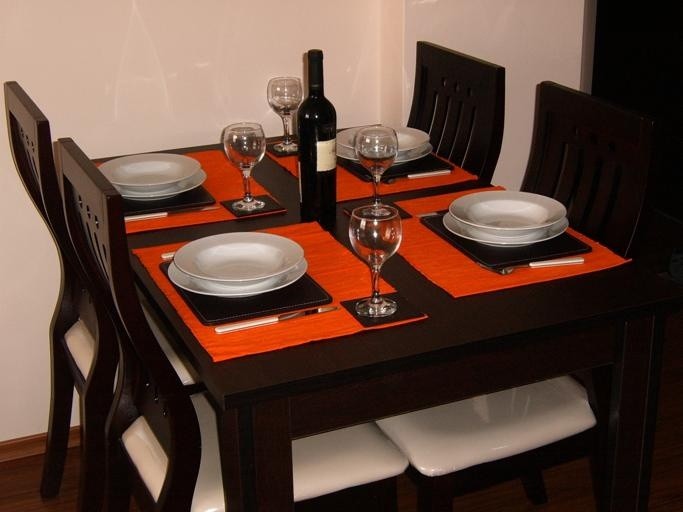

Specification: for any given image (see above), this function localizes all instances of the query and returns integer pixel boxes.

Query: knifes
[414,208,447,218]
[214,306,338,335]
[123,206,221,222]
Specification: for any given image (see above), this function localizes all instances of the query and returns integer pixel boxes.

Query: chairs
[1,79,205,511]
[404,40,506,184]
[47,130,410,511]
[371,79,666,511]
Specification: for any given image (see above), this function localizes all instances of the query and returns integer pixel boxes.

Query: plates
[335,124,429,152]
[110,168,206,202]
[172,232,303,286]
[166,256,308,298]
[442,211,569,249]
[448,191,566,236]
[97,152,201,193]
[335,142,434,166]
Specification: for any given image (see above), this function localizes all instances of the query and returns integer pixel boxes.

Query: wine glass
[348,202,403,318]
[223,121,267,211]
[355,123,399,218]
[266,76,302,154]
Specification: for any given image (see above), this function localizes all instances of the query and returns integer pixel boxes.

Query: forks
[364,168,451,182]
[476,256,585,276]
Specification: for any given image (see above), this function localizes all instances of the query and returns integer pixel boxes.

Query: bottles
[299,49,337,234]
